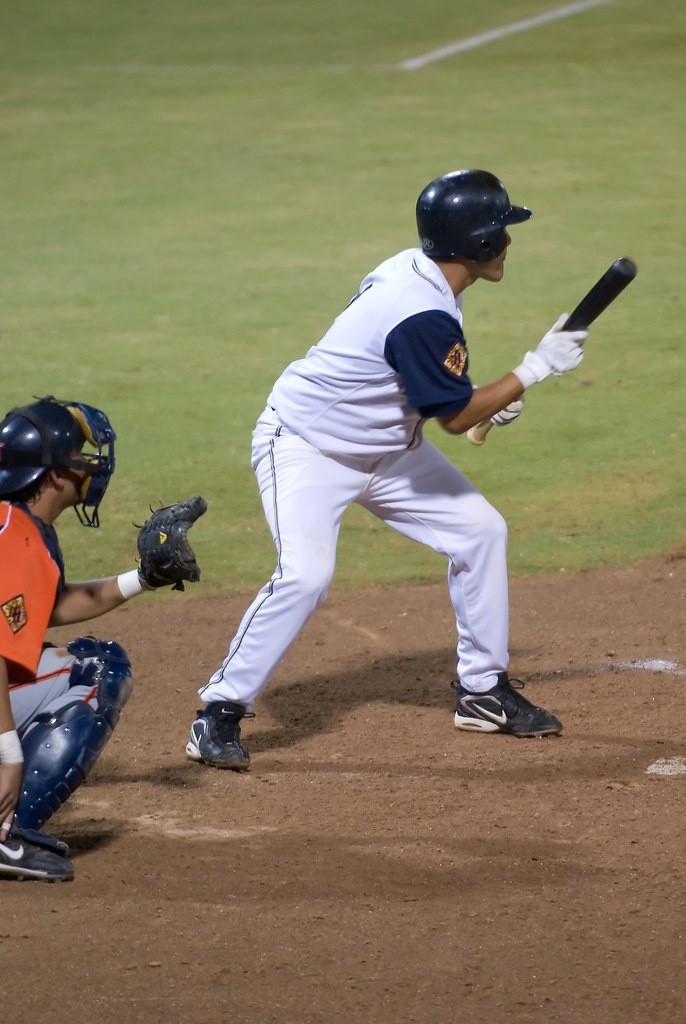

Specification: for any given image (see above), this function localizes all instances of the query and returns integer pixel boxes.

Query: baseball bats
[468,257,638,449]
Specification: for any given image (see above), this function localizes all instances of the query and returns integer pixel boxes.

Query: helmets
[417,168,533,261]
[2,396,90,496]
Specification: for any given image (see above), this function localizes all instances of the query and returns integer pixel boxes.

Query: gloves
[513,314,586,387]
[474,383,523,427]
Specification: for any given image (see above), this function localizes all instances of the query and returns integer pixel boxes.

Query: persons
[185,169,589,769]
[0,395,209,883]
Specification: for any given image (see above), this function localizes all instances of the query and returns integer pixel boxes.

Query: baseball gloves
[133,497,208,593]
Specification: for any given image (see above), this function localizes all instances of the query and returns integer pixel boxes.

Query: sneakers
[184,701,251,773]
[451,673,566,738]
[0,835,76,883]
